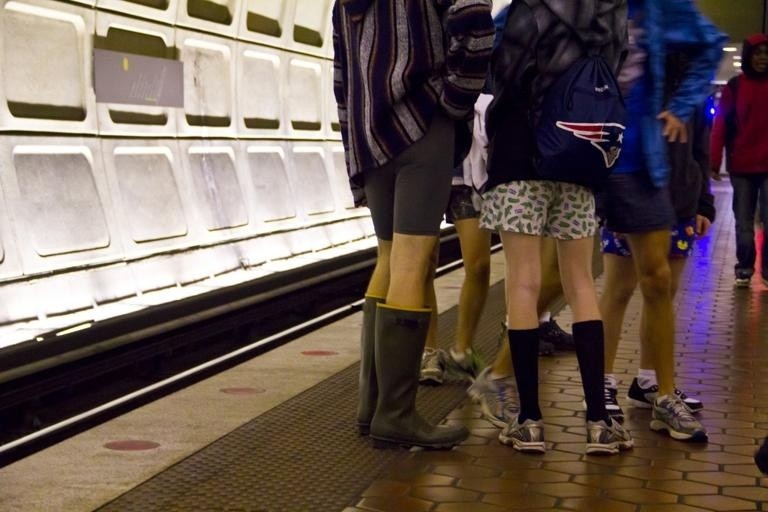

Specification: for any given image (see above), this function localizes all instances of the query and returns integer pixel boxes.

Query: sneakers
[736,272,751,287]
[419,318,708,455]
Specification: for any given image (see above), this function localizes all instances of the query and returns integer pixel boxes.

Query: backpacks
[526,0,627,182]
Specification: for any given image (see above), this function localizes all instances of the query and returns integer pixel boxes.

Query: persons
[331,0,768,459]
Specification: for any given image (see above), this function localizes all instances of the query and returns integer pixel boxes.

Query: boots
[356,294,469,450]
